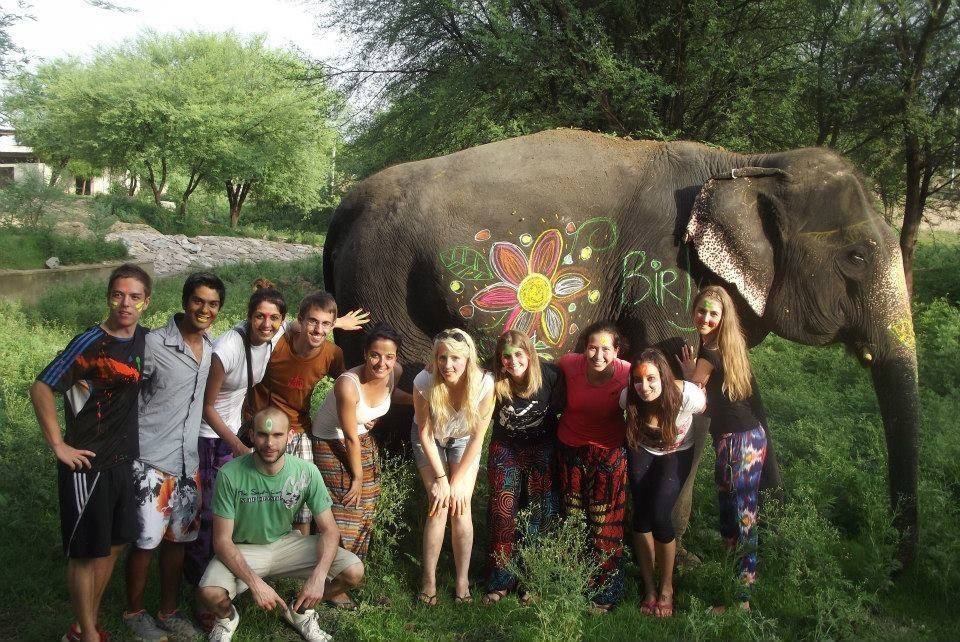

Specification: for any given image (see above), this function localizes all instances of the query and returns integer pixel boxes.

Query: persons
[675,285,767,614]
[410,328,496,605]
[198,407,364,642]
[311,324,413,613]
[237,290,378,535]
[122,273,225,642]
[554,323,632,615]
[30,264,152,579]
[183,279,370,634]
[483,330,566,609]
[619,349,707,618]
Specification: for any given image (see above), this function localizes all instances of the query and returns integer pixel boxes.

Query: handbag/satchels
[239,421,256,448]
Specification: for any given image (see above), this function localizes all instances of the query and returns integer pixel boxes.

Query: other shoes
[334,594,358,610]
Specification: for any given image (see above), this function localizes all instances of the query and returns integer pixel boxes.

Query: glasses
[437,332,470,348]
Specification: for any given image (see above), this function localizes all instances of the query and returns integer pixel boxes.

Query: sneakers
[62,624,108,642]
[155,611,198,637]
[123,609,168,642]
[208,604,240,642]
[285,599,335,642]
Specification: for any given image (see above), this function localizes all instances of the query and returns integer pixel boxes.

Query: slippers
[192,608,215,631]
[454,596,474,607]
[655,602,673,618]
[481,590,505,606]
[414,592,438,608]
[639,600,655,616]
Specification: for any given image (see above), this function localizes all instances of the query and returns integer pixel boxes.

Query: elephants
[321,129,919,588]
